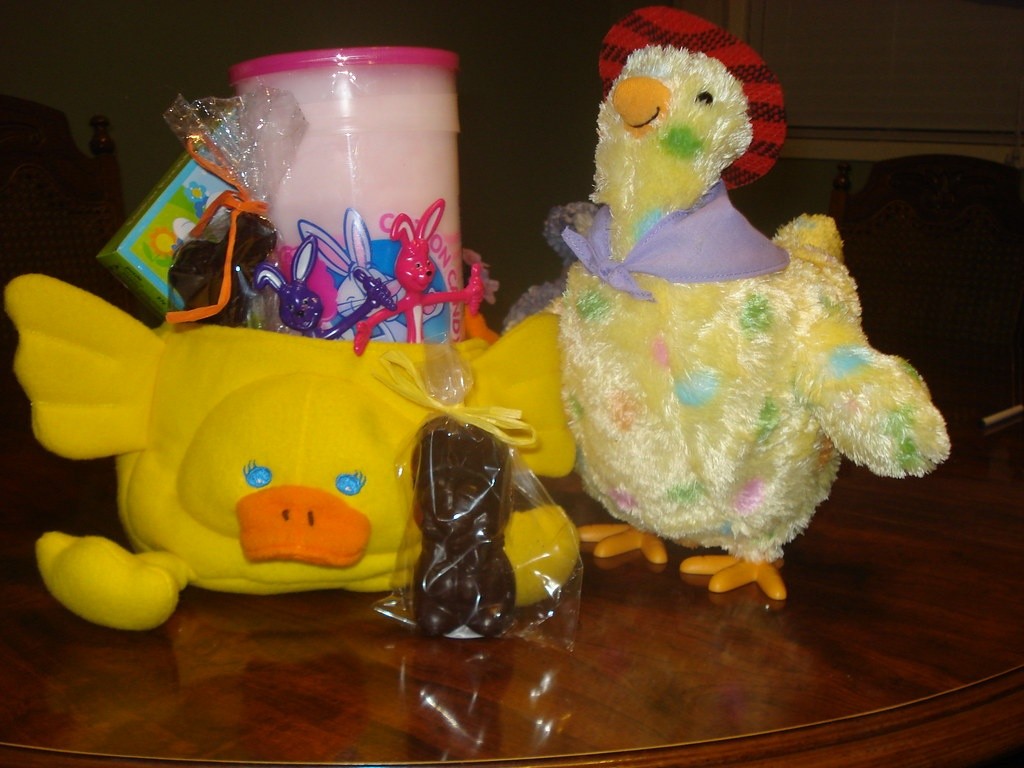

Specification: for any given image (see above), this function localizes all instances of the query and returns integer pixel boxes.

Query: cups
[226,46,465,350]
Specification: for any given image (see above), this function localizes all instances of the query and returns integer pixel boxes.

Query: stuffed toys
[7,271,581,632]
[501,6,950,599]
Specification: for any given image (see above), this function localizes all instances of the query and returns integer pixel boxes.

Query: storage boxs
[95,105,240,318]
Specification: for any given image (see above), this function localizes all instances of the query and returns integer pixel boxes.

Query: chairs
[0,91,140,355]
[828,151,1024,386]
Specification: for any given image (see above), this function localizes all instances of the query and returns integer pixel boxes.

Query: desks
[0,369,1024,768]
